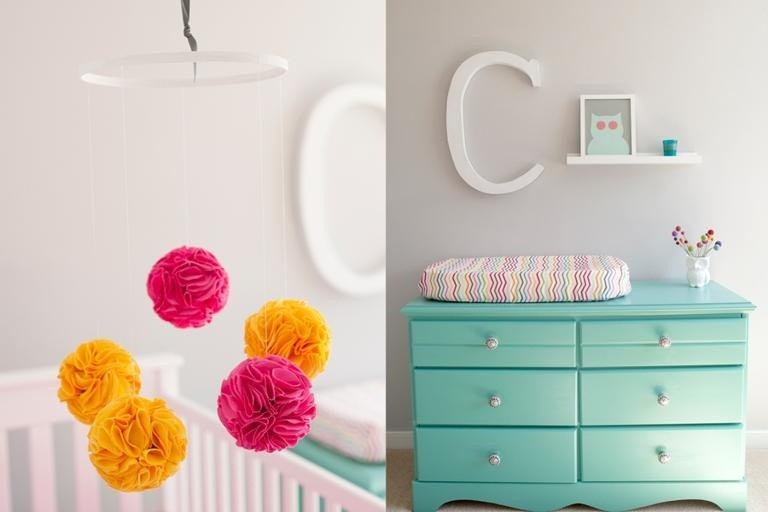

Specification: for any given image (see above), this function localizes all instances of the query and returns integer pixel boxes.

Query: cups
[663,139,678,157]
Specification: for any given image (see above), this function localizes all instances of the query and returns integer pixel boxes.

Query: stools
[286,436,387,512]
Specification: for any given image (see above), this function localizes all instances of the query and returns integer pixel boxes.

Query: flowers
[671,225,722,256]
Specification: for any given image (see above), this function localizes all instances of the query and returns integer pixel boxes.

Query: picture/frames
[580,95,636,157]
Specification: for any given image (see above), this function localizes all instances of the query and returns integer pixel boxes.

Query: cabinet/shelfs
[400,280,757,512]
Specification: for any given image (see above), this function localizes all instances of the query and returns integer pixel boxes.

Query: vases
[687,256,710,287]
